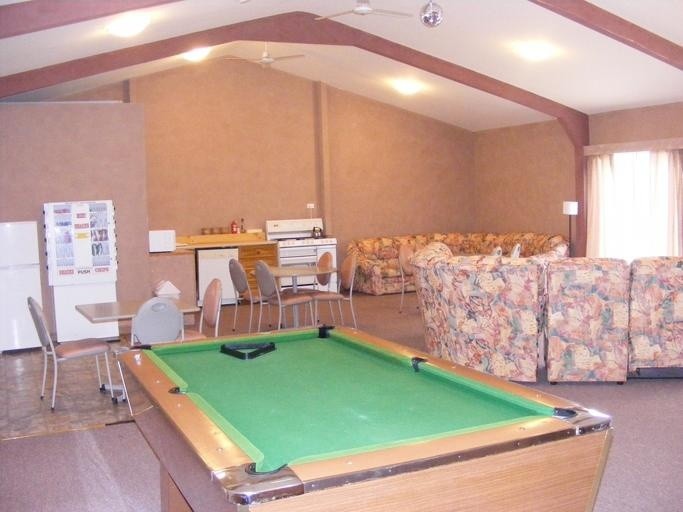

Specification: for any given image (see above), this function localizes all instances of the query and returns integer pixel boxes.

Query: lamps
[419,0,443,28]
[561,200,579,257]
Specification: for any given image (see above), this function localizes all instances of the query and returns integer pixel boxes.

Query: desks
[250,267,338,327]
[75,296,200,324]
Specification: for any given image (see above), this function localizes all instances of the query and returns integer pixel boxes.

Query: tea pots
[311,226,323,239]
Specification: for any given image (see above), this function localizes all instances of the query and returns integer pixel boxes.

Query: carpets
[0,327,207,443]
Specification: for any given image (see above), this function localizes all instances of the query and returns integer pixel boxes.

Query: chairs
[126,296,184,346]
[254,259,314,333]
[547,258,628,386]
[312,255,357,328]
[228,258,283,332]
[184,278,223,341]
[305,252,336,323]
[397,244,414,314]
[28,296,114,411]
[628,257,683,375]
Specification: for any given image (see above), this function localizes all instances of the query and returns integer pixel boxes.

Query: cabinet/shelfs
[239,244,278,302]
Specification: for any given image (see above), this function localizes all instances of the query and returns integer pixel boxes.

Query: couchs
[410,240,540,383]
[347,231,569,296]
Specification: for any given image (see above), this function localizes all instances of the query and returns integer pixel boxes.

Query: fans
[223,43,304,68]
[313,0,411,21]
